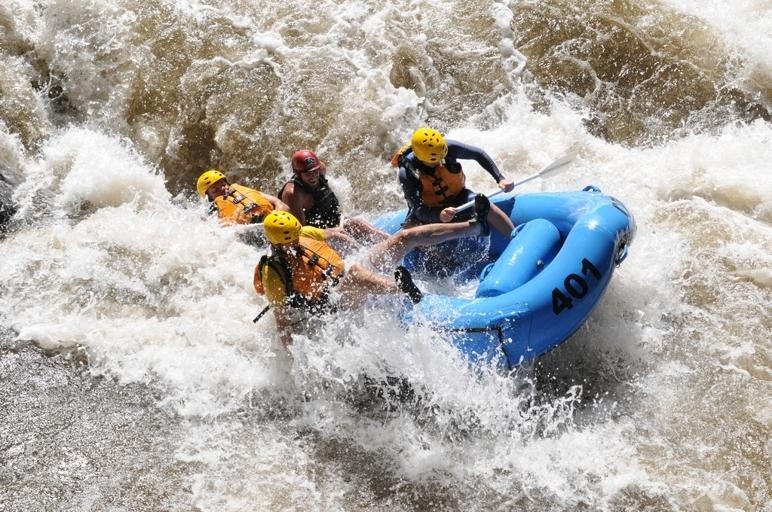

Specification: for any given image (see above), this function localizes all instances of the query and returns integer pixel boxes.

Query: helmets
[264,210,302,245]
[412,128,449,163]
[291,150,321,174]
[197,170,227,199]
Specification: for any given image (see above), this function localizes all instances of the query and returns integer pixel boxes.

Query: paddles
[453,156,574,213]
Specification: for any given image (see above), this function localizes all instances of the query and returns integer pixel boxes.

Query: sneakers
[469,193,491,237]
[394,264,422,304]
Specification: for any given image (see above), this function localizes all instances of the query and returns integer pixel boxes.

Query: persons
[261,194,490,360]
[398,128,515,273]
[277,150,392,259]
[196,169,290,250]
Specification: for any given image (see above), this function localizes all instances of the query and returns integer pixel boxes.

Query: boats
[319,184,636,383]
